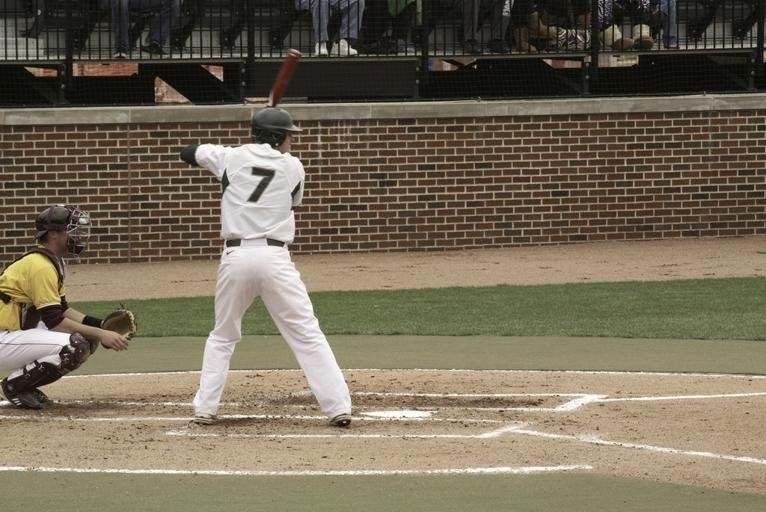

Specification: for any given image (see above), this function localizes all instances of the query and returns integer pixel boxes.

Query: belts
[226,238,284,246]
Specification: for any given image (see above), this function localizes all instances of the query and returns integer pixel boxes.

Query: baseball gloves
[97,306,138,349]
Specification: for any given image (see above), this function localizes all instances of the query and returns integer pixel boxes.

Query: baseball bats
[266,46,302,109]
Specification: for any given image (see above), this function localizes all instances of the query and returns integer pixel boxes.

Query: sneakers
[460,39,481,52]
[114,48,126,57]
[664,42,680,48]
[330,39,357,56]
[0,380,48,408]
[315,42,328,55]
[195,414,218,424]
[143,41,160,51]
[487,40,509,52]
[334,414,351,426]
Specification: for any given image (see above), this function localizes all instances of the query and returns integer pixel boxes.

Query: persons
[180,108,352,427]
[294,2,680,56]
[0,204,129,411]
[109,1,299,57]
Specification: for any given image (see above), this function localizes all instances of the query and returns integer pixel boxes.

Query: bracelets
[82,315,102,327]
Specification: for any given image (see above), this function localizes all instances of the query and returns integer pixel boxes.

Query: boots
[513,28,536,52]
[600,24,634,50]
[633,24,653,49]
[528,12,556,39]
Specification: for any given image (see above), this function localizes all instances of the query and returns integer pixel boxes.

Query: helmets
[35,204,91,256]
[251,109,303,142]
